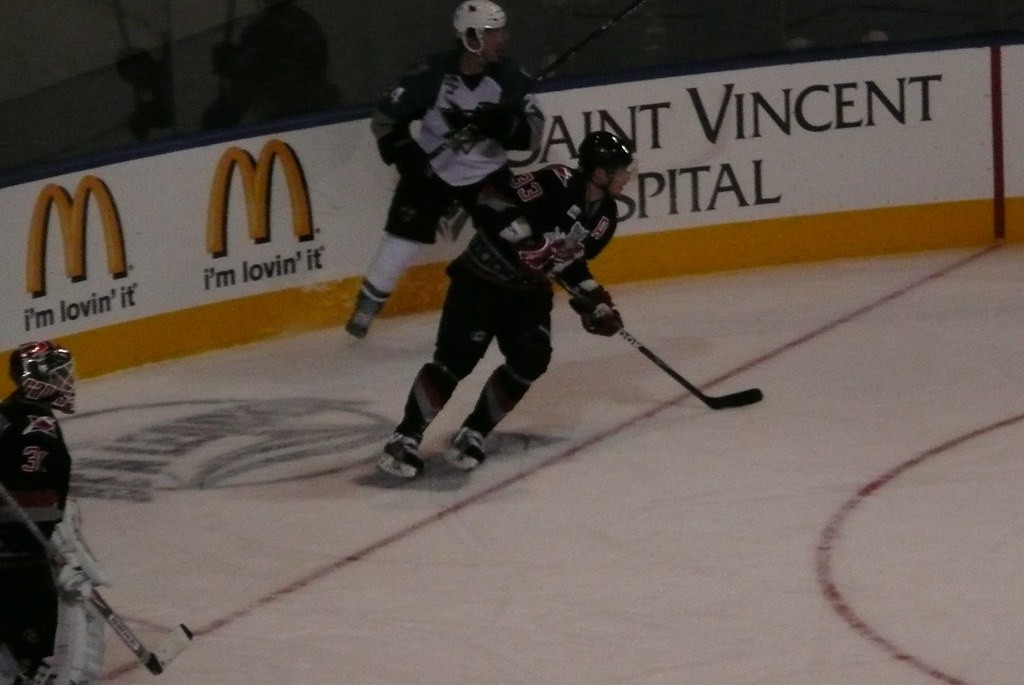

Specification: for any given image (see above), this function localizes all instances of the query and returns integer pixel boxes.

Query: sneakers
[346,309,374,344]
[446,427,485,472]
[377,431,424,478]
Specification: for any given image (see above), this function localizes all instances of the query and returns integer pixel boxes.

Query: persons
[0,340,94,685]
[383,129,638,468]
[344,0,546,339]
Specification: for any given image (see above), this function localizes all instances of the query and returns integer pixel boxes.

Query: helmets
[578,130,633,174]
[451,0,507,39]
[11,341,75,414]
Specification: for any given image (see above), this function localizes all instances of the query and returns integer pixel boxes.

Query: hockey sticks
[1,481,197,677]
[424,0,647,162]
[552,274,768,412]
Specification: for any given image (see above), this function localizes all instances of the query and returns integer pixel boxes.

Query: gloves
[569,284,624,336]
[473,101,528,145]
[394,142,431,183]
[517,236,555,279]
